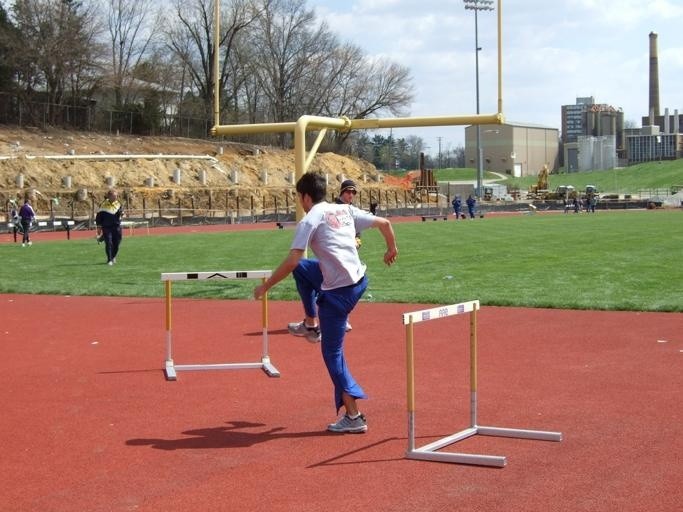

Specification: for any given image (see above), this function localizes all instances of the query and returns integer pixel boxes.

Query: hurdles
[161,271,280,381]
[402,300,563,467]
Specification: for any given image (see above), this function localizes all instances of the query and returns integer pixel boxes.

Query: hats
[340,180,354,193]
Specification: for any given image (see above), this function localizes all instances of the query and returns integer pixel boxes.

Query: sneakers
[327,412,367,433]
[287,319,321,343]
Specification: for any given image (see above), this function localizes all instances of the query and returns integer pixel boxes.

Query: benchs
[422,215,447,221]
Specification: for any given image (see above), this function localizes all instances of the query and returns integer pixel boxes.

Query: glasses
[344,190,356,194]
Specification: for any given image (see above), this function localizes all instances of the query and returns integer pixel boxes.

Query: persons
[19,199,35,247]
[452,194,476,220]
[96,188,124,266]
[334,180,362,332]
[253,171,398,433]
[563,194,597,214]
